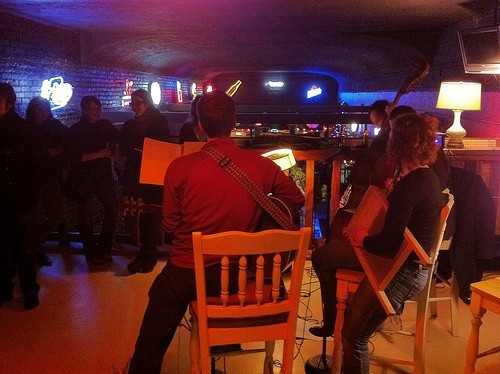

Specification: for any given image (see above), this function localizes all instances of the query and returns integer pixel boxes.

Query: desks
[229,136,500,230]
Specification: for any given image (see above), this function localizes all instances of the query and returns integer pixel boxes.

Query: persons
[308,100,457,374]
[116,89,169,276]
[126,91,304,374]
[0,82,120,312]
[179,94,207,144]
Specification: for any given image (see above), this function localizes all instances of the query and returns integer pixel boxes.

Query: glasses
[129,100,146,107]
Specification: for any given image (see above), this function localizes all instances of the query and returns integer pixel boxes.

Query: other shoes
[127,259,153,274]
[24,295,40,309]
[85,251,113,264]
[38,253,53,265]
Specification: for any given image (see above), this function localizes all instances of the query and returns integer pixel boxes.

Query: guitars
[112,195,300,280]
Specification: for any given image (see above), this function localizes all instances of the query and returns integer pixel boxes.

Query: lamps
[436,82,482,149]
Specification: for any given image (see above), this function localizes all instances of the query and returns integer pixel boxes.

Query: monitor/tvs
[457,25,500,74]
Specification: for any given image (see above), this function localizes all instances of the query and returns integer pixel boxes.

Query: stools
[466,277,500,374]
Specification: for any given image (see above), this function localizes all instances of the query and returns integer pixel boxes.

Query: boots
[308,297,338,338]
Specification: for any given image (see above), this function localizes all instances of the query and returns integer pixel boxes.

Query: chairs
[335,189,454,374]
[188,227,312,374]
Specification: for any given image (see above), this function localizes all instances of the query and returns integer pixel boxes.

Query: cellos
[325,56,429,245]
